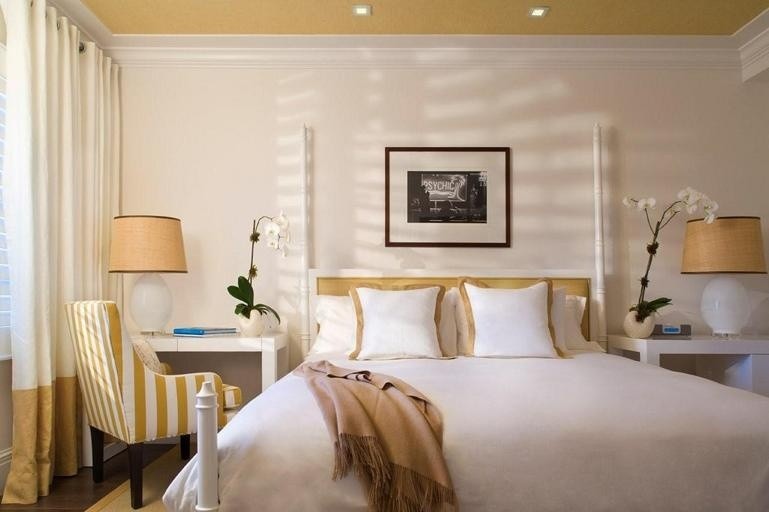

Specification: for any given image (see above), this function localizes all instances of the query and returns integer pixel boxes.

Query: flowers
[225,208,292,326]
[621,185,720,322]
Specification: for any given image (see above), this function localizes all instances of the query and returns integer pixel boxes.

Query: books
[173,333,241,338]
[174,327,236,335]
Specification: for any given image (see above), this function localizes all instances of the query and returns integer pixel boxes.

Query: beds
[160,117,769,512]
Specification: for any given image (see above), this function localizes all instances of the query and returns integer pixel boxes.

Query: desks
[141,334,290,393]
[608,333,769,400]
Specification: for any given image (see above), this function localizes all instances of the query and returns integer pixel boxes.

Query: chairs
[64,299,242,509]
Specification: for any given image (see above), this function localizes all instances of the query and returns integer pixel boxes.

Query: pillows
[459,277,564,359]
[129,337,162,375]
[306,285,459,361]
[565,293,597,350]
[345,281,445,360]
[453,286,569,358]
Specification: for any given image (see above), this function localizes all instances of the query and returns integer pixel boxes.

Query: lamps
[681,216,769,337]
[108,215,189,336]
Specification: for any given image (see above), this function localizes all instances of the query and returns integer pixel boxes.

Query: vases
[623,310,656,339]
[237,310,267,338]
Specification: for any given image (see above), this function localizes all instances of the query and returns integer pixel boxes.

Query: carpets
[83,442,199,512]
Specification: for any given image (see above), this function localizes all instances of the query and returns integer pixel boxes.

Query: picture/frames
[384,146,512,248]
